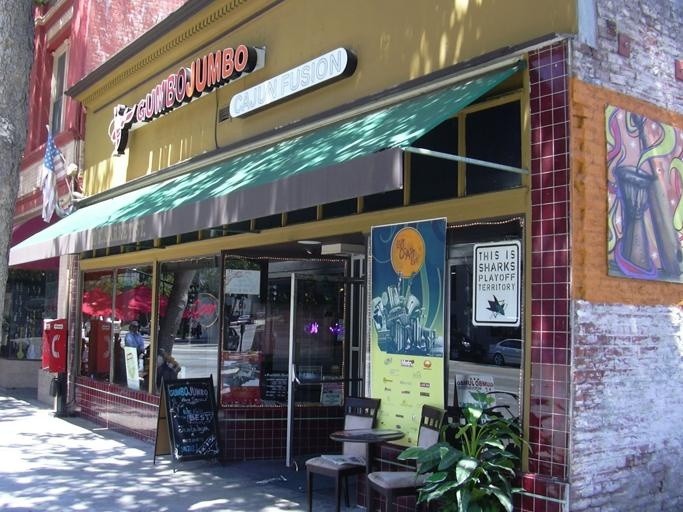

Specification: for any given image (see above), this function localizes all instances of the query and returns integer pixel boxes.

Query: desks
[330,428,405,511]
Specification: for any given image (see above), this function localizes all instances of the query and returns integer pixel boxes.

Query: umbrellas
[79,283,170,324]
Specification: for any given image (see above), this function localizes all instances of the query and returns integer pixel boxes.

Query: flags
[33,130,64,224]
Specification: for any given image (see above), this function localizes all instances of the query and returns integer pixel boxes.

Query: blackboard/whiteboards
[163,378,222,462]
[259,369,288,403]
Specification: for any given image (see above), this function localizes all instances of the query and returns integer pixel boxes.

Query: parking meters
[239,323,246,352]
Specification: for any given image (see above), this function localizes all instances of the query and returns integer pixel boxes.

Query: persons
[79,322,181,394]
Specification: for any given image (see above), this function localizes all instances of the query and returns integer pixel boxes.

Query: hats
[130,320,140,327]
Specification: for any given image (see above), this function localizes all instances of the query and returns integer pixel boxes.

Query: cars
[227,328,240,350]
[236,314,254,326]
[483,338,528,370]
[449,330,472,362]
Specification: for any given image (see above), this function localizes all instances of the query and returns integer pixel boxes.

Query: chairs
[368,404,445,511]
[305,396,382,512]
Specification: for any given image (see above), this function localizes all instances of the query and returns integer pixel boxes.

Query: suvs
[83,311,123,340]
